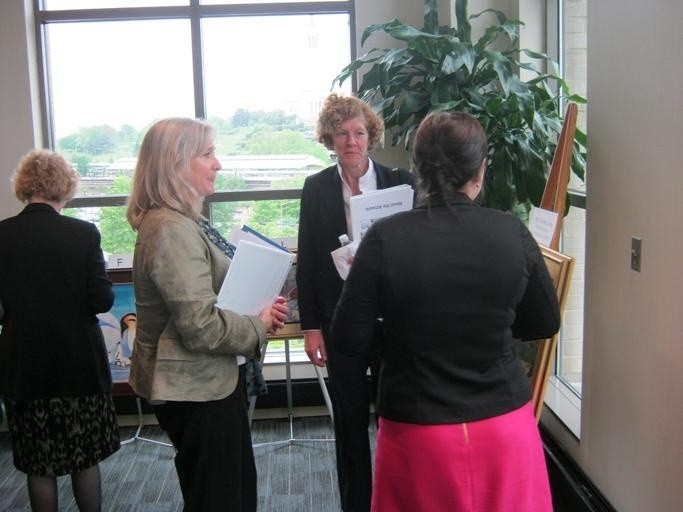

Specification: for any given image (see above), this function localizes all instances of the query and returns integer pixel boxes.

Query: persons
[0,149,121,512]
[126,118,289,512]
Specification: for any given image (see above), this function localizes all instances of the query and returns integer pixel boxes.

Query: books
[214,224,296,318]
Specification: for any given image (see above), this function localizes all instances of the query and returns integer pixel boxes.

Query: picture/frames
[513,244,577,426]
[96,269,157,397]
